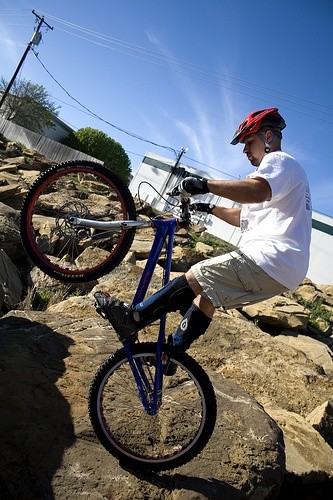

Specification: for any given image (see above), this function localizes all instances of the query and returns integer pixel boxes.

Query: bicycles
[18,158,219,472]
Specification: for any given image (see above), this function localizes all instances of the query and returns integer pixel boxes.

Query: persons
[93,107,313,376]
